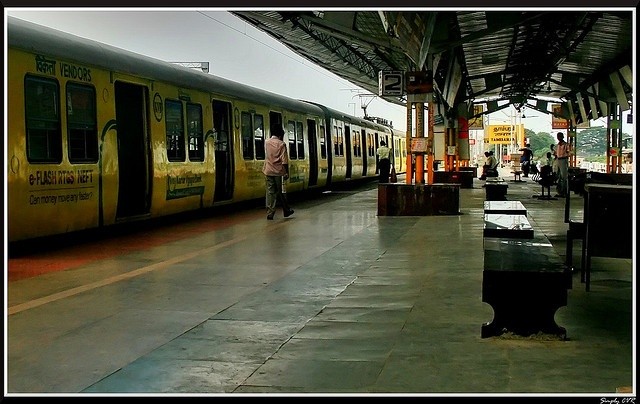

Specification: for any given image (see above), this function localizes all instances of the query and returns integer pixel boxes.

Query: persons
[376,141,390,183]
[625,154,633,172]
[552,132,570,198]
[490,151,496,164]
[479,151,496,180]
[547,152,553,166]
[550,144,558,172]
[519,144,533,177]
[262,125,294,219]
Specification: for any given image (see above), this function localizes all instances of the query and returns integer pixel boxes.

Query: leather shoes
[267,215,273,220]
[284,210,294,217]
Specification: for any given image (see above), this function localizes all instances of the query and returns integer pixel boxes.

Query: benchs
[482,182,508,200]
[486,177,504,182]
[484,201,527,218]
[481,237,568,337]
[483,214,533,239]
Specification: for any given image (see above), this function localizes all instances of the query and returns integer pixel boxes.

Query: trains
[8,15,408,245]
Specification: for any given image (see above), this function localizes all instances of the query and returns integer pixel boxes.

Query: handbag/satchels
[391,164,397,183]
